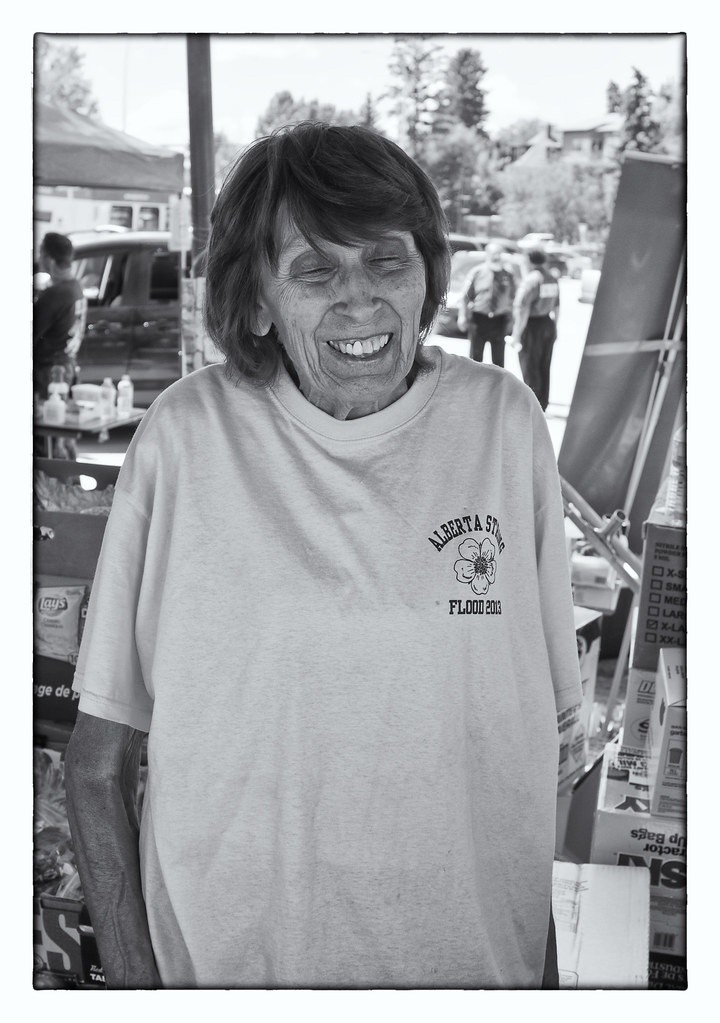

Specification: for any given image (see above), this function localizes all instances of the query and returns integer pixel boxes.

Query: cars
[59,226,133,251]
[430,232,606,340]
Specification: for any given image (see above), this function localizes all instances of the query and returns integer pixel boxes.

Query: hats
[528,249,546,264]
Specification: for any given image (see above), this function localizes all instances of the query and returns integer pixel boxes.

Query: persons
[65,119,587,989]
[32,232,88,461]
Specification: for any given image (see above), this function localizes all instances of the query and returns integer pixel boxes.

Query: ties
[488,272,500,317]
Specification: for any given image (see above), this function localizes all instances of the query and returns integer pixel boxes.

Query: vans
[35,231,184,414]
